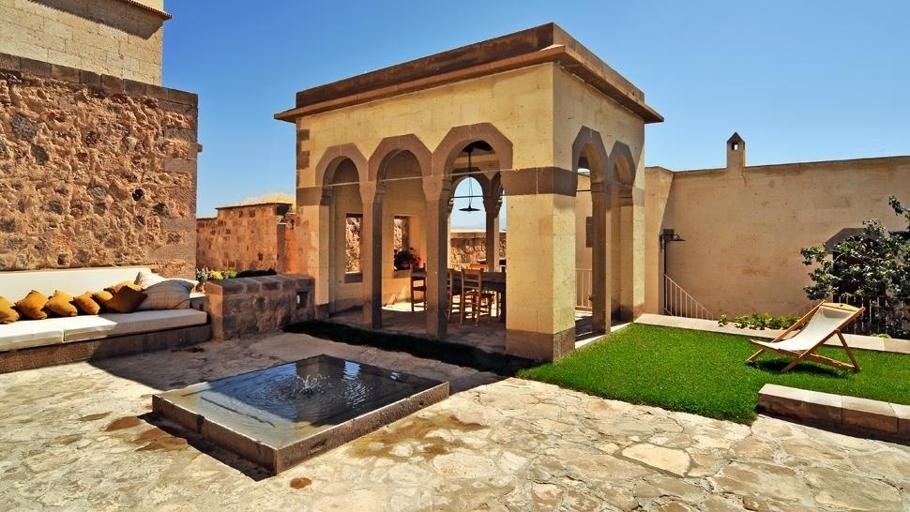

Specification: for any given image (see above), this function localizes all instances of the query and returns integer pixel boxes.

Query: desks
[414,270,506,323]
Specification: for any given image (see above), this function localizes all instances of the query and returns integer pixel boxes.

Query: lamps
[458,146,481,213]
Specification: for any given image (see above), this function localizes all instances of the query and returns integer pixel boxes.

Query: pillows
[0,270,199,322]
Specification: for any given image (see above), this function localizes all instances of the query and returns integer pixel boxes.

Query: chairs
[745,299,867,374]
[407,262,492,328]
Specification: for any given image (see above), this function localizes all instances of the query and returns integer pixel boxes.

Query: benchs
[1,263,210,372]
